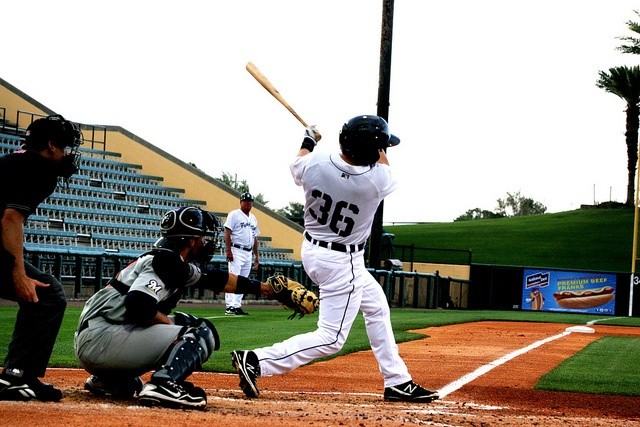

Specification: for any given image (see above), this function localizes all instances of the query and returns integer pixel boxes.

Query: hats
[240,193,254,202]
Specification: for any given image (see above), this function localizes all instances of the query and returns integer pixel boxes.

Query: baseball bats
[246,62,321,139]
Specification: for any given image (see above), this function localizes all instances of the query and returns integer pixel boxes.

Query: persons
[0,114,85,400]
[223,192,261,314]
[74,205,288,407]
[230,114,440,403]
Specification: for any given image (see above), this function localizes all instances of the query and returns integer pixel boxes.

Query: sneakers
[384,380,439,402]
[0,368,63,401]
[229,350,260,398]
[235,308,249,315]
[84,375,143,398]
[225,306,239,315]
[133,379,207,408]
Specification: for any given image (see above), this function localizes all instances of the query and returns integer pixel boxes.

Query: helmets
[153,205,222,276]
[339,115,400,166]
[25,114,84,189]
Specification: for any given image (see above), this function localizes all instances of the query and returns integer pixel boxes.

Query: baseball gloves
[268,275,319,320]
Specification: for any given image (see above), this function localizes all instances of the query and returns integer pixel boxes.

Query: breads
[553,285,614,309]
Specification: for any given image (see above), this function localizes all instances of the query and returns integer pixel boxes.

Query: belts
[231,242,252,252]
[305,231,365,252]
